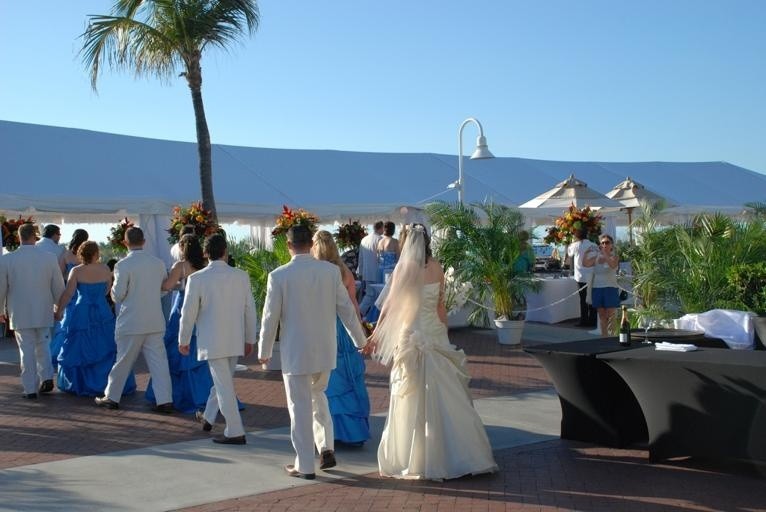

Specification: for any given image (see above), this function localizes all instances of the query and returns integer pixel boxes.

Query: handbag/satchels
[586,268,594,305]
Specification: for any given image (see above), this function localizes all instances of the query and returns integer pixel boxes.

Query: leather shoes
[319,450,336,469]
[22,393,37,398]
[153,402,173,412]
[196,410,212,431]
[285,465,315,479]
[94,396,118,408]
[213,434,245,444]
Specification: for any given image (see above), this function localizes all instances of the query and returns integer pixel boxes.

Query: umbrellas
[590,177,682,242]
[518,173,627,213]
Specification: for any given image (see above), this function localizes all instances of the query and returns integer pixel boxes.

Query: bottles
[619,305,632,348]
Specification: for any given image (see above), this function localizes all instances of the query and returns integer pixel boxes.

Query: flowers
[0,216,42,252]
[108,218,137,254]
[164,201,222,245]
[270,205,318,235]
[543,204,605,247]
[334,217,368,250]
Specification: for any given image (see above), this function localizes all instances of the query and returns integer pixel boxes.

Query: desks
[352,268,636,329]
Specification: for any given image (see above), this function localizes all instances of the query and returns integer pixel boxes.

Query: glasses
[602,241,609,244]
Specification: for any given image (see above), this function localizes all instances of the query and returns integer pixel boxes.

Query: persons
[258,219,498,482]
[0,223,257,445]
[566,219,619,336]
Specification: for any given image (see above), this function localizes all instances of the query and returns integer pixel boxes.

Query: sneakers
[40,380,53,392]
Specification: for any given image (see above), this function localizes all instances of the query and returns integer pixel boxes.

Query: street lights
[450,115,494,224]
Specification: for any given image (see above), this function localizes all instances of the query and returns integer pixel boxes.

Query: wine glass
[640,315,653,344]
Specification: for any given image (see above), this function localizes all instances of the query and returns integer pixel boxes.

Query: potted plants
[424,195,528,345]
